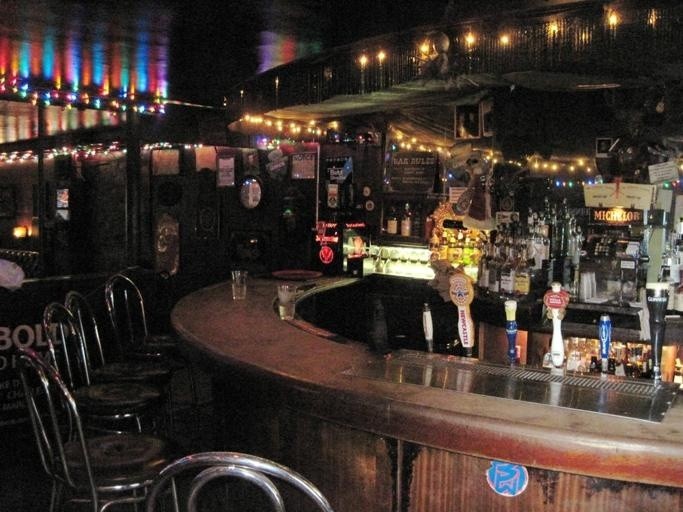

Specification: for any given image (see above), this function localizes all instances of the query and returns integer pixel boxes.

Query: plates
[271,269,323,280]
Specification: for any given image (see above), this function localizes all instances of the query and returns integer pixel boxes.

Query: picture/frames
[215,154,238,189]
[54,187,71,210]
[453,101,481,140]
[482,97,495,138]
[386,151,438,194]
[241,148,260,176]
[149,147,183,177]
[289,151,317,181]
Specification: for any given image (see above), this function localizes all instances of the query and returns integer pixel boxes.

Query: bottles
[439,198,585,300]
[543,338,657,376]
[386,203,423,238]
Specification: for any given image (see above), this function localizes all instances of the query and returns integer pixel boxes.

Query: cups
[232,271,247,301]
[278,285,296,320]
[660,345,678,383]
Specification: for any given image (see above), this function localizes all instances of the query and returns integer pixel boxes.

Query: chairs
[143,452,335,511]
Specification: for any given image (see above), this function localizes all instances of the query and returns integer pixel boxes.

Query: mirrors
[237,174,264,212]
